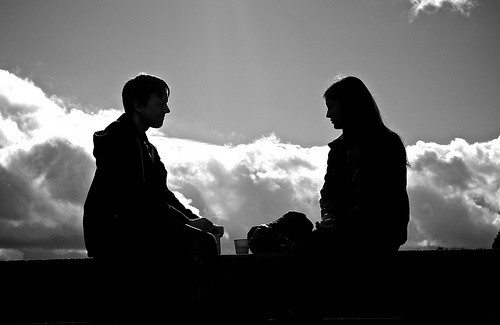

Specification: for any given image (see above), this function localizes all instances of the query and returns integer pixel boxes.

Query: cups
[234,238,250,256]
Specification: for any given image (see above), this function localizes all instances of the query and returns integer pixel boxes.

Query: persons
[83,73,223,259]
[300,76,410,256]
